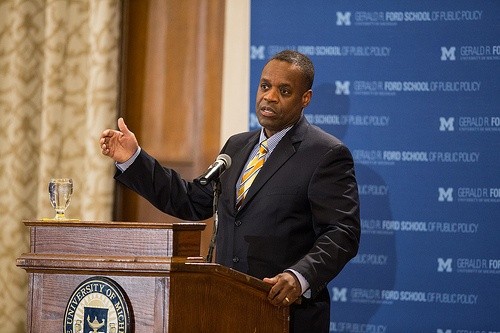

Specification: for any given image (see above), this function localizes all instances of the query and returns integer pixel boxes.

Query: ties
[235,140,269,213]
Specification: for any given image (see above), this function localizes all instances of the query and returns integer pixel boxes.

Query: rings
[285,298,290,303]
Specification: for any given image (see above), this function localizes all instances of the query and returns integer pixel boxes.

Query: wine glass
[48,178,73,220]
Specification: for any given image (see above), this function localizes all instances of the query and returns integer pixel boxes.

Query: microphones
[199,154,231,187]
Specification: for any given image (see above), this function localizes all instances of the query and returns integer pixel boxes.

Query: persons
[99,50,362,333]
